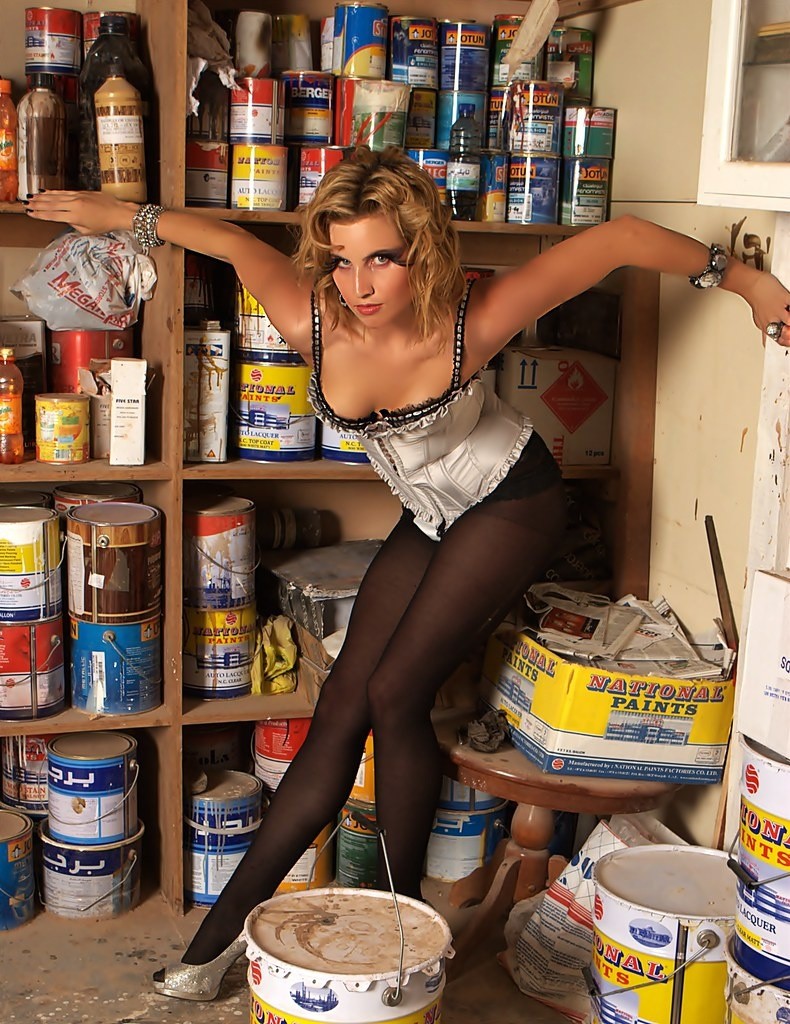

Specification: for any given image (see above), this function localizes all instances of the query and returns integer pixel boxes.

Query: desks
[430,705,682,976]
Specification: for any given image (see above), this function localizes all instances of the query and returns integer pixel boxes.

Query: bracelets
[132,203,165,249]
[690,240,732,295]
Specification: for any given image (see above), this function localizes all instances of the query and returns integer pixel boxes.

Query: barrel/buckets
[231,272,375,466]
[0,485,790,1023]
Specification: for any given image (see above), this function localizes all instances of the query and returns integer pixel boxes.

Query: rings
[766,321,785,342]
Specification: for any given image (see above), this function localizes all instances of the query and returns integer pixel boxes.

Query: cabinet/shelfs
[0,0,662,918]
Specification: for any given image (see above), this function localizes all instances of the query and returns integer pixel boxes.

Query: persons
[22,146,790,1003]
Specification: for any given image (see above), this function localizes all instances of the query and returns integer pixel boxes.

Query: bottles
[77,17,150,196]
[95,61,149,204]
[0,77,20,204]
[447,103,483,222]
[0,347,25,464]
[12,73,66,202]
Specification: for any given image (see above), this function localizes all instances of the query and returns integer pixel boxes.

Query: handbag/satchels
[10,228,157,331]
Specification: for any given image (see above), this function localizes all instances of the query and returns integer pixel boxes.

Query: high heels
[155,922,248,1002]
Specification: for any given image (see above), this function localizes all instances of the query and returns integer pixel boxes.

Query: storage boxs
[738,569,790,762]
[478,630,736,785]
[79,356,156,465]
[498,345,617,465]
[257,538,385,707]
